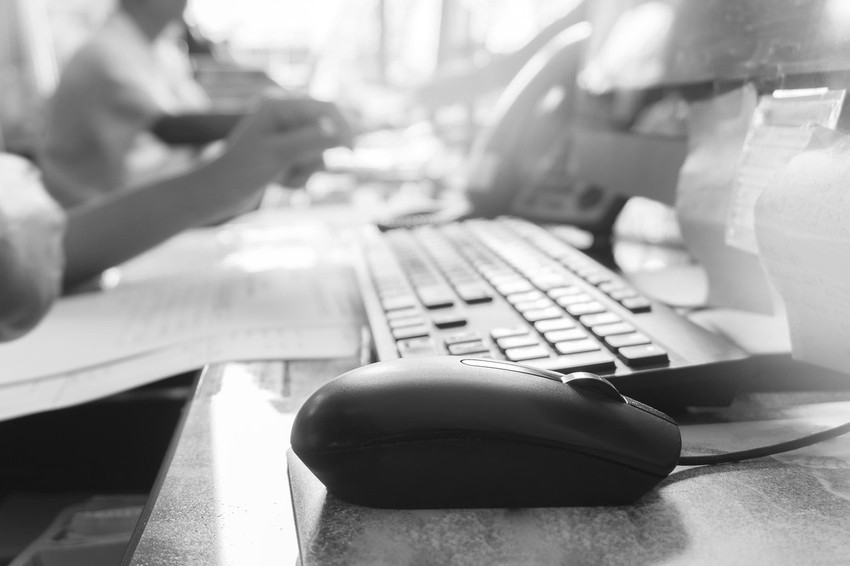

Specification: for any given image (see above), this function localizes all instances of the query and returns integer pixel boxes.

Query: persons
[39,0,245,193]
[0,92,358,349]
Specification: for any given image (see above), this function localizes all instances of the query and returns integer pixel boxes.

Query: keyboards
[356,208,752,409]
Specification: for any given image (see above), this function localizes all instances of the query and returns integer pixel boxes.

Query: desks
[122,358,850,566]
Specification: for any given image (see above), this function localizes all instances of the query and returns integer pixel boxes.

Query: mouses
[290,354,680,512]
